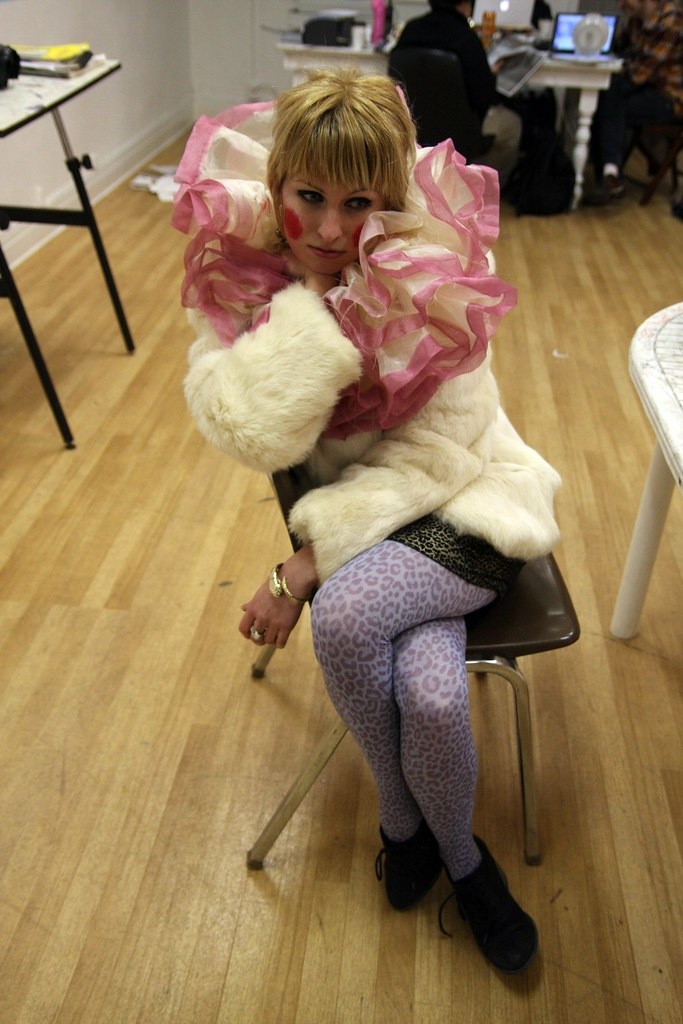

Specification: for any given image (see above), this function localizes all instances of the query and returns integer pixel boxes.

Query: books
[487,36,548,96]
[9,42,107,79]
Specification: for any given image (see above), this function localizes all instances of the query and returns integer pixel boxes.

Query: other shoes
[604,176,624,197]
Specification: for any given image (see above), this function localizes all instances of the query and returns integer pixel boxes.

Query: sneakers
[375,818,443,912]
[438,836,538,972]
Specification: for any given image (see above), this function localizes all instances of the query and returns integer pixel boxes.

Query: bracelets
[269,563,311,603]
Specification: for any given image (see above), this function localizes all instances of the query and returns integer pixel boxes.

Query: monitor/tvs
[552,12,619,55]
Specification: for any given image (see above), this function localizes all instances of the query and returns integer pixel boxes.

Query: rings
[249,628,262,641]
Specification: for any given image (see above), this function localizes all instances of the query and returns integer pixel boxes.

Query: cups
[349,25,365,49]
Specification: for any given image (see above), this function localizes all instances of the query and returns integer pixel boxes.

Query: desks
[610,302,683,639]
[277,42,623,210]
[0,61,134,451]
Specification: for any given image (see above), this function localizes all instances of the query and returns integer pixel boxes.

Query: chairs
[620,123,682,205]
[387,47,495,166]
[247,465,580,871]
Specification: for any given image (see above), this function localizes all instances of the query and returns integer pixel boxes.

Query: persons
[388,0,521,186]
[580,0,683,206]
[171,70,563,974]
